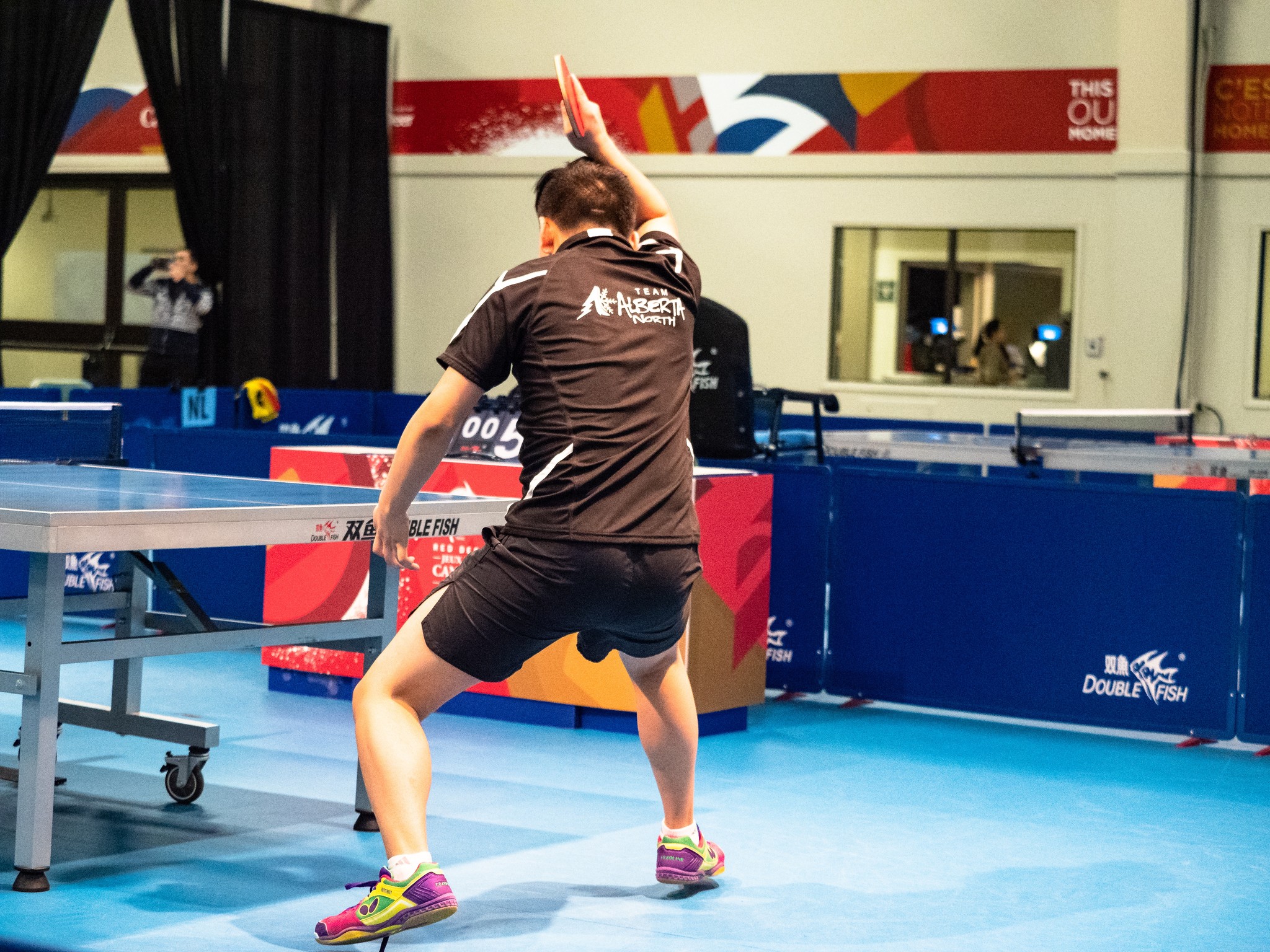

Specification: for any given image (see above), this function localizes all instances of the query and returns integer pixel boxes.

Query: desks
[0,459,521,893]
[262,443,769,740]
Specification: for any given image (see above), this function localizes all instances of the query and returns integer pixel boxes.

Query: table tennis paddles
[553,55,585,138]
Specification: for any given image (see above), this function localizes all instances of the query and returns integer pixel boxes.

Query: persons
[126,249,214,389]
[974,315,1012,384]
[314,75,724,945]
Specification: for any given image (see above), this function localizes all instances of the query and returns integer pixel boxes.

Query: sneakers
[655,824,727,883]
[315,858,455,944]
